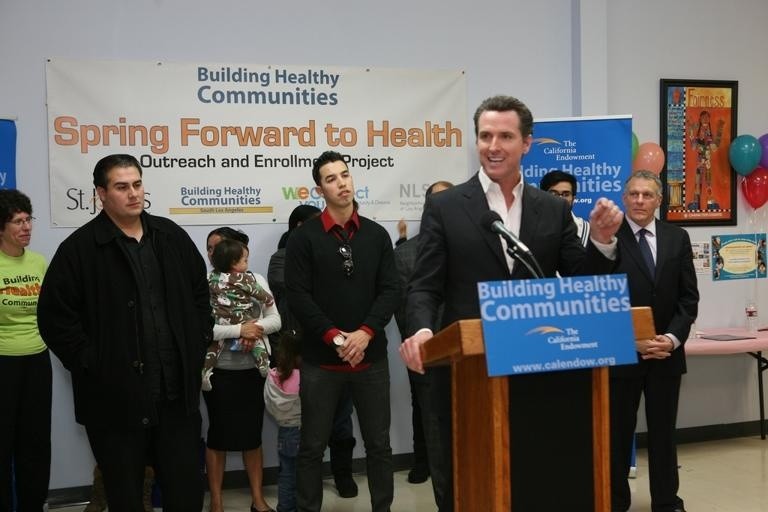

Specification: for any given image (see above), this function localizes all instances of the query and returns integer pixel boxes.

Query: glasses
[339,244,354,277]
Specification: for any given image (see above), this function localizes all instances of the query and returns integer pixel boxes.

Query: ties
[639,229,655,278]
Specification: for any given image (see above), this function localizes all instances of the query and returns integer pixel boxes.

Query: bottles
[743,295,759,337]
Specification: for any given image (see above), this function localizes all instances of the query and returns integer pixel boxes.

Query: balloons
[727,133,768,211]
[632,132,665,173]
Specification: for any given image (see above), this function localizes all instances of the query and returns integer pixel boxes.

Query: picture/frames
[660,79,738,227]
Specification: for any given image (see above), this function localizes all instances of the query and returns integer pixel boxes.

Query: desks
[683,327,768,440]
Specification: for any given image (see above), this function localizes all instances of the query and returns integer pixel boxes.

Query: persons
[0,187,53,511]
[267,150,397,510]
[395,182,452,483]
[399,95,625,375]
[713,236,767,279]
[36,154,215,511]
[540,171,590,247]
[609,172,698,512]
[201,227,283,510]
[686,109,725,210]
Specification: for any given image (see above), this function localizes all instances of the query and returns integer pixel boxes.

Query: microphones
[478,208,530,255]
